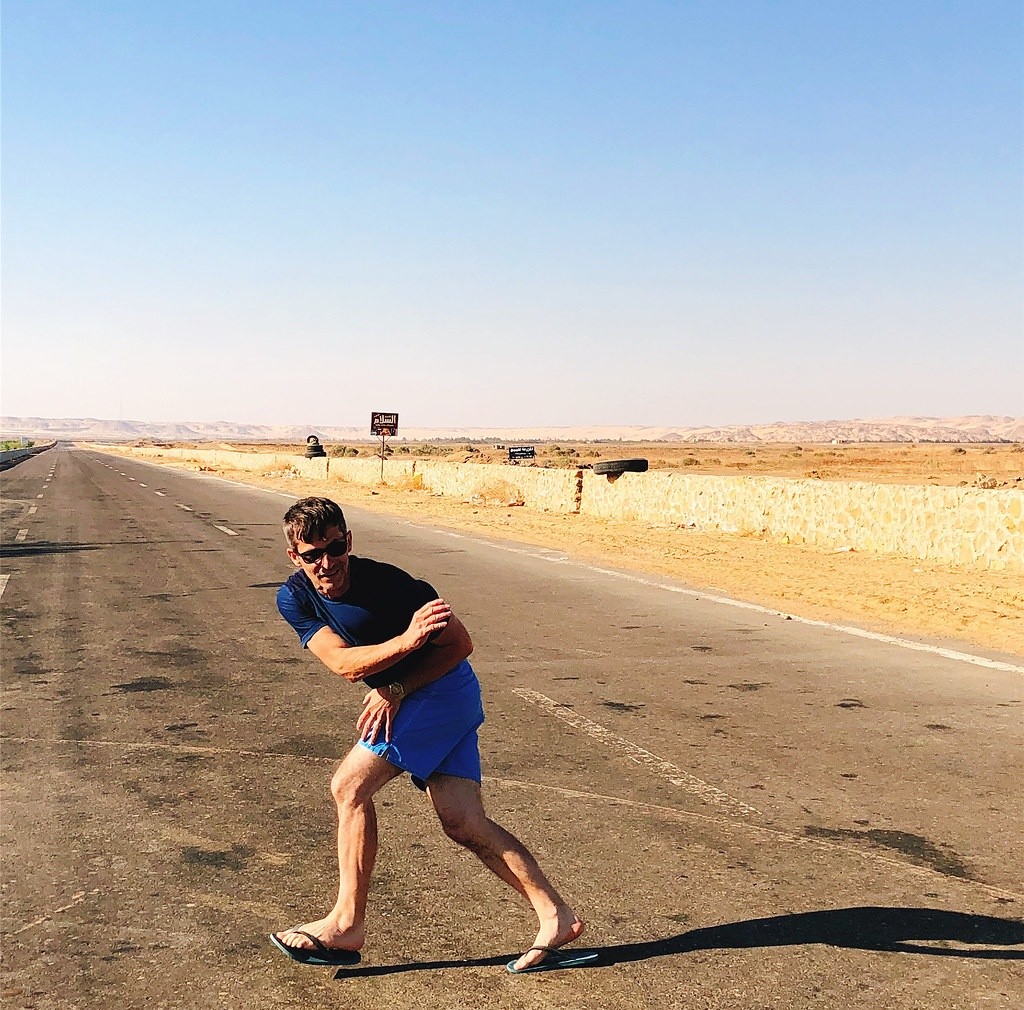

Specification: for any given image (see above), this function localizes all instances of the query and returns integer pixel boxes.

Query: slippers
[270,923,362,966]
[507,945,599,974]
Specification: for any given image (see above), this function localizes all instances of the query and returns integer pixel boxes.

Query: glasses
[294,534,349,564]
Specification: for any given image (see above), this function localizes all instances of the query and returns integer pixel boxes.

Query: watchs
[388,682,405,699]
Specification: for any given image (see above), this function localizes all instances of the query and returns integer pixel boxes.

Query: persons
[269,497,599,973]
